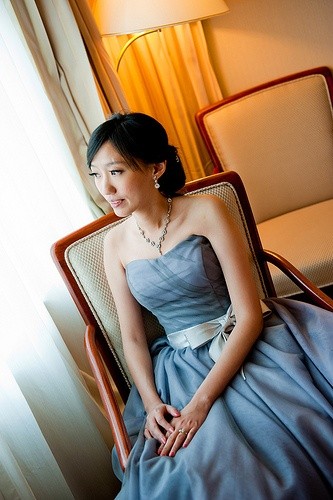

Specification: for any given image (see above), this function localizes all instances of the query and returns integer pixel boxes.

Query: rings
[178,430,187,435]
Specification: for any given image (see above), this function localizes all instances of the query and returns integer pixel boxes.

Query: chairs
[49,169,333,475]
[193,66,333,298]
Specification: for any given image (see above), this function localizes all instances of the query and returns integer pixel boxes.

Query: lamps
[91,0,231,75]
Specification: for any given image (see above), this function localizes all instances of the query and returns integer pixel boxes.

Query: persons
[86,112,333,500]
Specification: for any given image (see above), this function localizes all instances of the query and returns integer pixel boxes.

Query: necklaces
[134,195,172,256]
